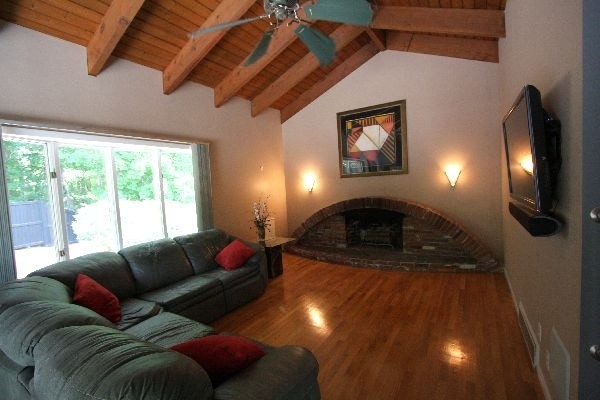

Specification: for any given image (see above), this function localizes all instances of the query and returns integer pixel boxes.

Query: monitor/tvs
[501,84,567,238]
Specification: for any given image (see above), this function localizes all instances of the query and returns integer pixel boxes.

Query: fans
[190,0,375,70]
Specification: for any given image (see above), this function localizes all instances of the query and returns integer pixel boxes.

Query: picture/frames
[335,98,408,178]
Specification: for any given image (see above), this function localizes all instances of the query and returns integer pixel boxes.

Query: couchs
[25,309,322,400]
[117,227,267,323]
[0,250,165,399]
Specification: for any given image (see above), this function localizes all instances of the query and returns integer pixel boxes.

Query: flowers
[246,192,275,236]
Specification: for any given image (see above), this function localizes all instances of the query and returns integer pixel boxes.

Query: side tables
[251,236,298,279]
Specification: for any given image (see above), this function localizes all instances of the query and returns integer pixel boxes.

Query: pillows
[214,238,258,271]
[170,334,270,377]
[72,271,122,325]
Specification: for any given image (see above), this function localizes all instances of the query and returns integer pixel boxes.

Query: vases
[257,228,266,246]
